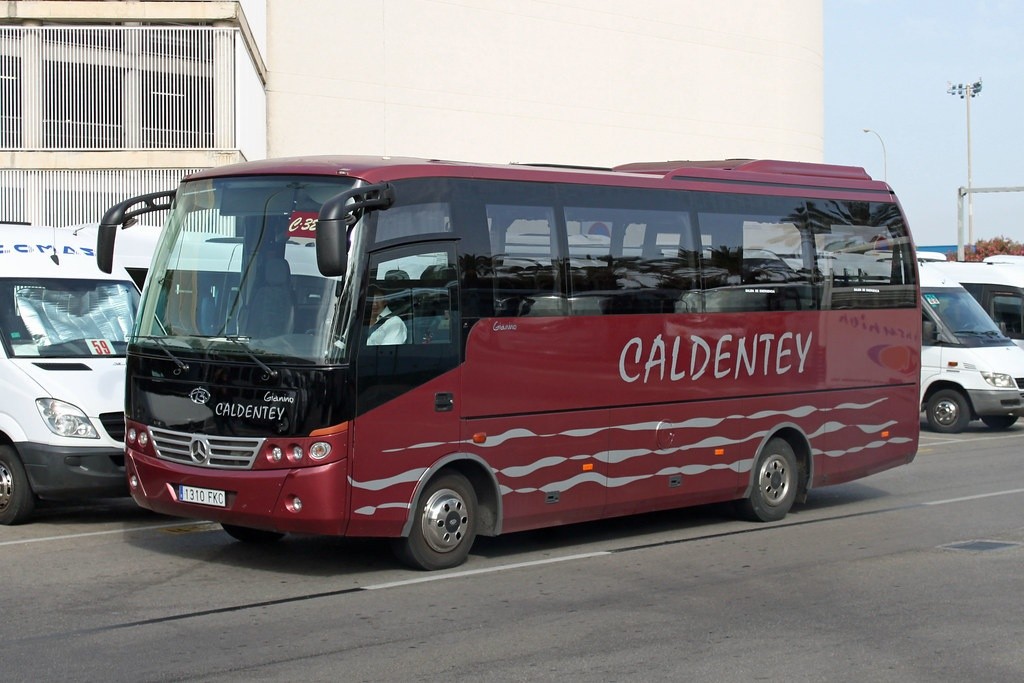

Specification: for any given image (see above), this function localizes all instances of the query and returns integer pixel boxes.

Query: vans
[763,244,1024,433]
[0,222,615,525]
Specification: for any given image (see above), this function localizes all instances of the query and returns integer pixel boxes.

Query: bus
[96,152,923,574]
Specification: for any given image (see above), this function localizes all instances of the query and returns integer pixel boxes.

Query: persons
[366,283,408,346]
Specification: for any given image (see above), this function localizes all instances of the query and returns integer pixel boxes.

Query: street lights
[863,127,888,183]
[946,79,982,260]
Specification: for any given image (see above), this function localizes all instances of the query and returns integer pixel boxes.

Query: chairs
[384,255,722,292]
[243,258,298,340]
